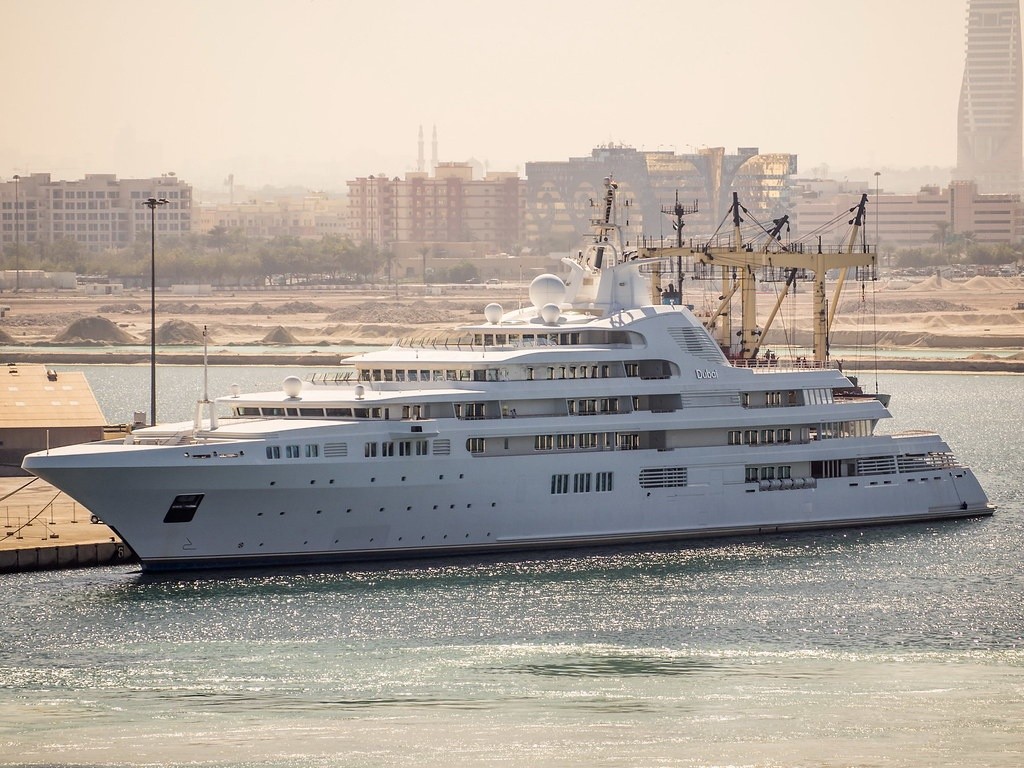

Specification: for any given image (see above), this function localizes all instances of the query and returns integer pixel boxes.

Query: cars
[880,264,1018,277]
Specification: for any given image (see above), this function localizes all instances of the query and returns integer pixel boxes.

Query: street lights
[12,175,21,289]
[142,196,172,428]
[874,172,881,245]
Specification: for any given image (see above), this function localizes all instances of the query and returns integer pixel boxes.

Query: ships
[19,236,997,574]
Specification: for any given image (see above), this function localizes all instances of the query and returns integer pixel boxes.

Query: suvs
[486,279,500,284]
[466,277,481,284]
